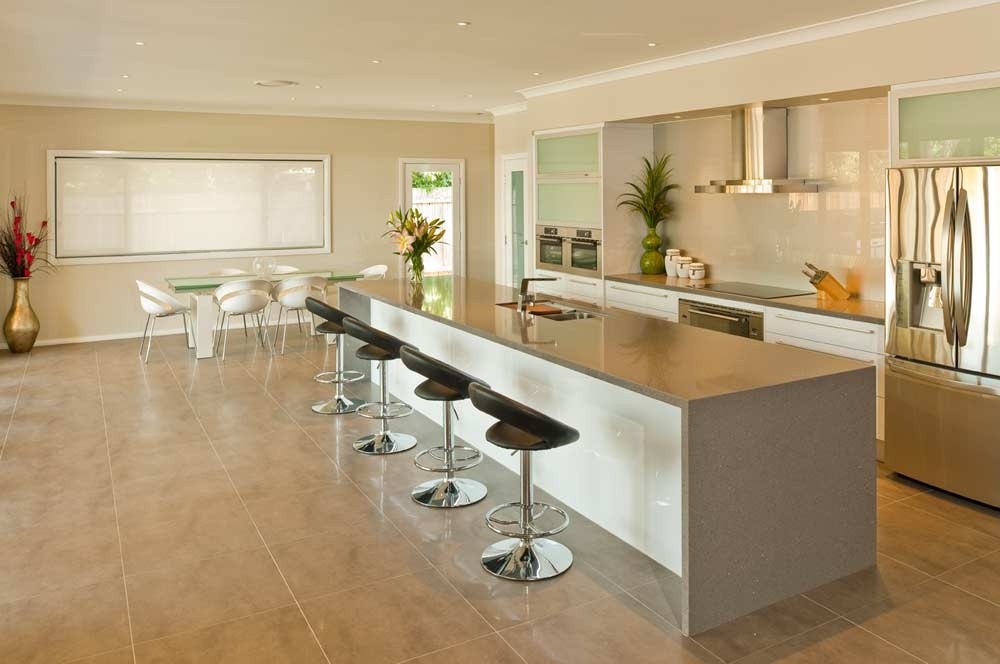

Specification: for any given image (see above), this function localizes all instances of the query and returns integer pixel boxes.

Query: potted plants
[615,151,681,274]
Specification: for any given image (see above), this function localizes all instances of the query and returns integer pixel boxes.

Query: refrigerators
[883,163,1000,510]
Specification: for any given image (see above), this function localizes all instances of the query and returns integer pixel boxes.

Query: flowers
[379,206,445,281]
[0,185,60,277]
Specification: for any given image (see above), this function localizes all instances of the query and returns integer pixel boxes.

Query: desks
[162,272,364,356]
[337,275,876,638]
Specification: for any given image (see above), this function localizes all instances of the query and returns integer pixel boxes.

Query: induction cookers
[685,282,816,299]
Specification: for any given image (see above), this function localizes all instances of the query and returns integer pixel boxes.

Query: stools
[305,297,580,581]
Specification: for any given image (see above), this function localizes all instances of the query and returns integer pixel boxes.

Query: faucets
[517,278,557,312]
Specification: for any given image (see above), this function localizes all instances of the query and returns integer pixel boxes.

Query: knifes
[802,262,819,279]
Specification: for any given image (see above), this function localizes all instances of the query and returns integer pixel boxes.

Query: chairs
[136,265,388,363]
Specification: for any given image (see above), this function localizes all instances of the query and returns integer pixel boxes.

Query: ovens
[535,225,602,280]
[679,299,764,342]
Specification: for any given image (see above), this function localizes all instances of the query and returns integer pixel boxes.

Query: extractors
[694,108,819,195]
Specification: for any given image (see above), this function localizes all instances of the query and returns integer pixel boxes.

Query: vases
[3,275,40,354]
[411,256,423,284]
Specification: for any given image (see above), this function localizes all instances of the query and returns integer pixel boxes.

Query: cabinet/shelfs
[536,269,602,307]
[534,120,654,227]
[605,280,678,322]
[763,307,885,463]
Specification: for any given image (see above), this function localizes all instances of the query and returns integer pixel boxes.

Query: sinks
[496,300,575,316]
[541,312,597,321]
